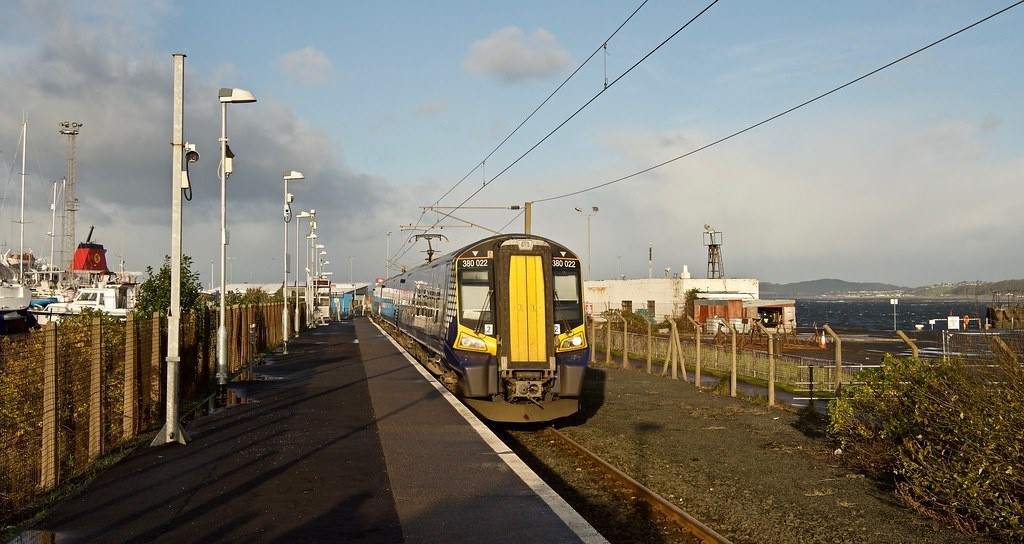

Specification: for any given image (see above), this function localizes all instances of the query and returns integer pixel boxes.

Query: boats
[25,288,144,329]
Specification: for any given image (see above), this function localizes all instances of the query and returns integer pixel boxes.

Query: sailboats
[0,108,72,317]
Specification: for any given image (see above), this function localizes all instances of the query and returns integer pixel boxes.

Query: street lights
[281,171,305,355]
[215,87,257,385]
[295,211,311,338]
[316,245,324,309]
[306,233,318,331]
[319,251,330,278]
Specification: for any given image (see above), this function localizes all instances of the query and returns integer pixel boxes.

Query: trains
[345,233,590,423]
